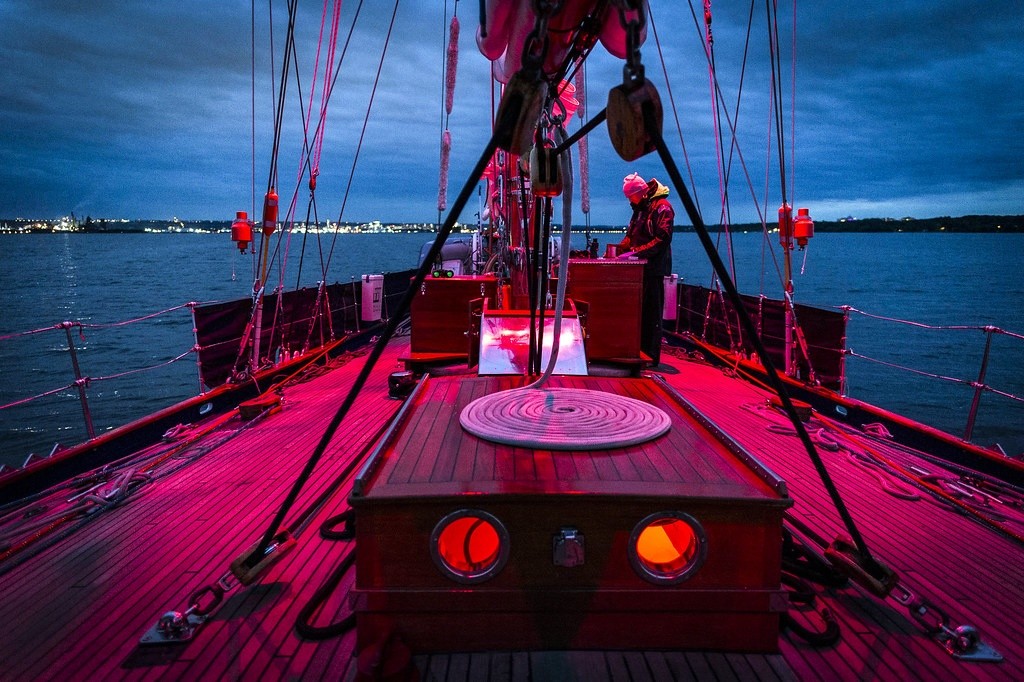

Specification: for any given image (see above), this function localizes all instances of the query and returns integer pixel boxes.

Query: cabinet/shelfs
[397,257,654,372]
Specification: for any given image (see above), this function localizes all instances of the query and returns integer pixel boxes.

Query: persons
[603,171,675,369]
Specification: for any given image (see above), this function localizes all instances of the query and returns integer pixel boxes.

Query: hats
[622,172,649,198]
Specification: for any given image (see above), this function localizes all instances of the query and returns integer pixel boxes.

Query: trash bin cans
[361,273,384,321]
[663,273,678,319]
